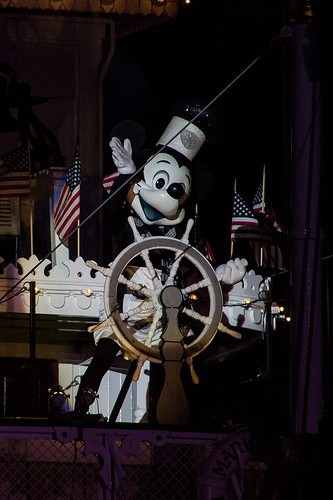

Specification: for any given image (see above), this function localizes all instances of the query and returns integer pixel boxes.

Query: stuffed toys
[70,105,246,424]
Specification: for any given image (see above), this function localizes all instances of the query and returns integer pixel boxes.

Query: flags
[101,171,121,195]
[0,146,32,200]
[250,184,286,272]
[52,133,81,242]
[231,190,273,245]
[194,215,214,265]
